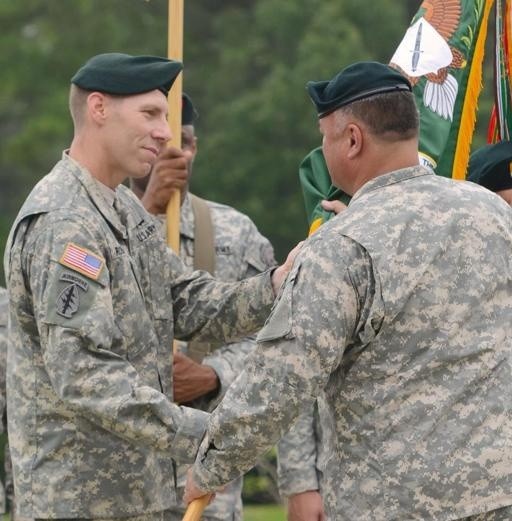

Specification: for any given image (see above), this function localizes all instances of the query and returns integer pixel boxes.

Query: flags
[297,0,496,228]
[487,0,512,144]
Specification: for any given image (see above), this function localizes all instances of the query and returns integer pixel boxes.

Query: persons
[3,52,307,521]
[180,59,512,521]
[0,285,15,521]
[277,398,328,521]
[465,142,512,208]
[129,90,280,521]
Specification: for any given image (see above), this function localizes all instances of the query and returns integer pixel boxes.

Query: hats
[182,92,199,124]
[305,61,413,118]
[70,53,184,95]
[465,141,512,192]
